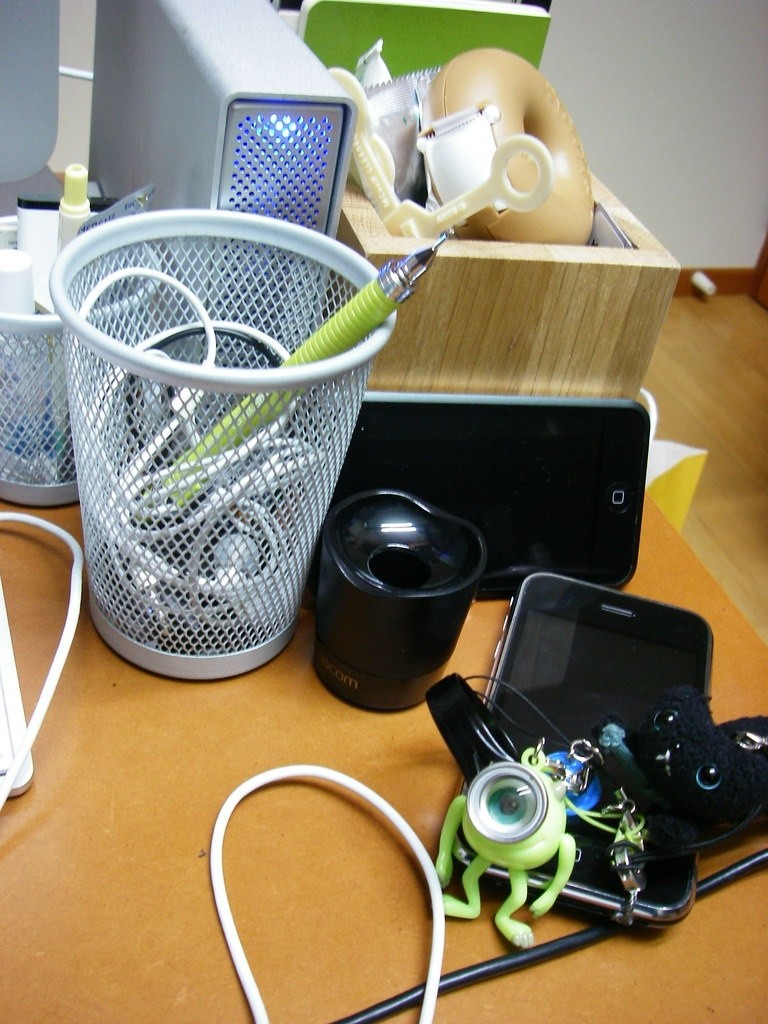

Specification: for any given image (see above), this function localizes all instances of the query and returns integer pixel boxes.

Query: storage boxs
[323,165,680,400]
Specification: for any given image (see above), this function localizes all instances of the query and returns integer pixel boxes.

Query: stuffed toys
[639,685,768,827]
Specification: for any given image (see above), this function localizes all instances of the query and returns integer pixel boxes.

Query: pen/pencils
[123,227,457,521]
[50,164,96,452]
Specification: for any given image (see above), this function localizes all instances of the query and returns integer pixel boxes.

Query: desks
[0,483,768,1022]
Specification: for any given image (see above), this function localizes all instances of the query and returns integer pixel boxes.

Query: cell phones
[307,391,651,593]
[450,571,714,924]
